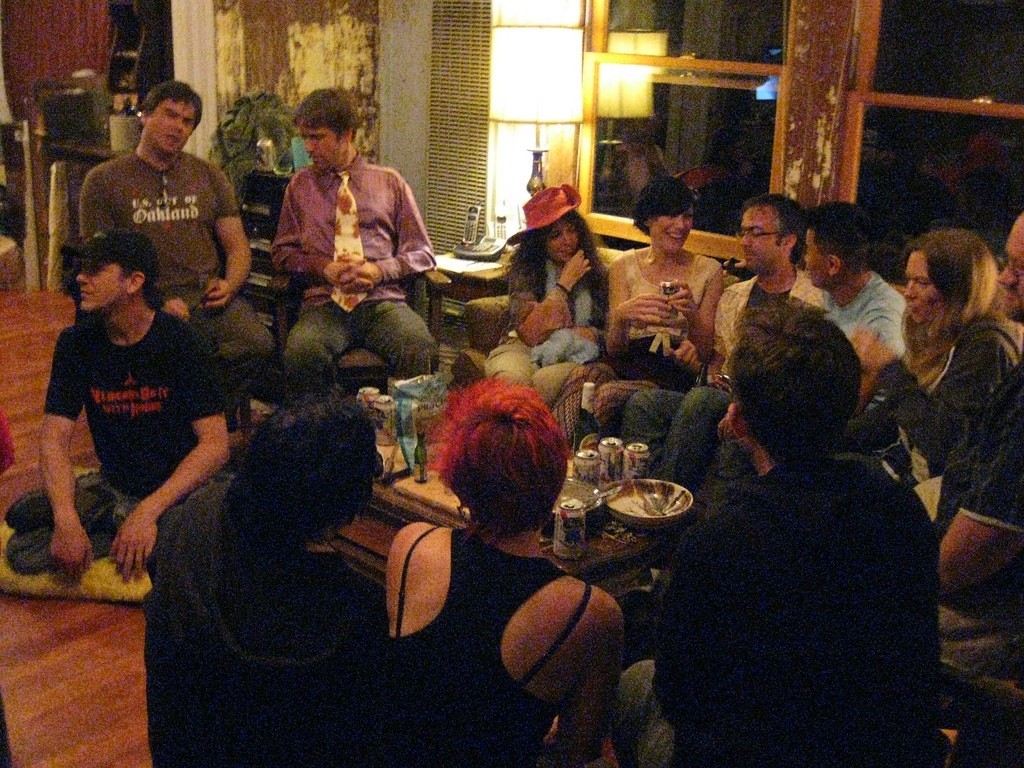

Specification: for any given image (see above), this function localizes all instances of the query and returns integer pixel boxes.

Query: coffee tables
[326,429,705,601]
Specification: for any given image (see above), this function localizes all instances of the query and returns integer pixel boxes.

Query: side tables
[434,252,514,301]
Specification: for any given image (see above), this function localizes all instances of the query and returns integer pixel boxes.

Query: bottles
[414,431,428,482]
[692,363,708,387]
[573,382,600,456]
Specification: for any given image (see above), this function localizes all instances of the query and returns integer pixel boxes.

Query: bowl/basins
[603,478,693,528]
[552,477,602,513]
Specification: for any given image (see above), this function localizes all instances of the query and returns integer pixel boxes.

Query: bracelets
[555,283,571,297]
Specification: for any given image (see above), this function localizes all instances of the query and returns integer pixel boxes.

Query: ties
[331,172,368,313]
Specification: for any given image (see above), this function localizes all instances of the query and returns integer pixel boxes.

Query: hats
[506,183,582,245]
[60,224,159,278]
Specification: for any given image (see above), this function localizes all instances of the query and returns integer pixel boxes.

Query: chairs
[268,270,452,391]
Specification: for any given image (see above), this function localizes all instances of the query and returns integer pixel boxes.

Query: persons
[79,81,275,372]
[848,227,1024,490]
[609,308,939,768]
[804,199,906,412]
[485,184,609,407]
[145,390,389,768]
[550,176,724,444]
[936,209,1024,682]
[623,193,824,514]
[383,378,623,768]
[271,88,436,398]
[4,228,230,582]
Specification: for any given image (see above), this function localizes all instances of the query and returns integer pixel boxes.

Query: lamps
[491,23,588,197]
[598,30,669,210]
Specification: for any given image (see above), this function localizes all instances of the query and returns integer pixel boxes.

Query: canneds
[572,437,650,489]
[659,281,679,319]
[356,386,398,446]
[553,499,586,560]
[256,138,274,171]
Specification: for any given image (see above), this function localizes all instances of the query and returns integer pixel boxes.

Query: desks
[15,119,121,295]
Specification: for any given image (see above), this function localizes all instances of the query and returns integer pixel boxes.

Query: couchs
[454,245,1024,385]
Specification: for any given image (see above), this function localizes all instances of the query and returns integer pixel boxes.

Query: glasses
[734,227,781,243]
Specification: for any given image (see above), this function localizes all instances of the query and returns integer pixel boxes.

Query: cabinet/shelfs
[219,172,292,323]
